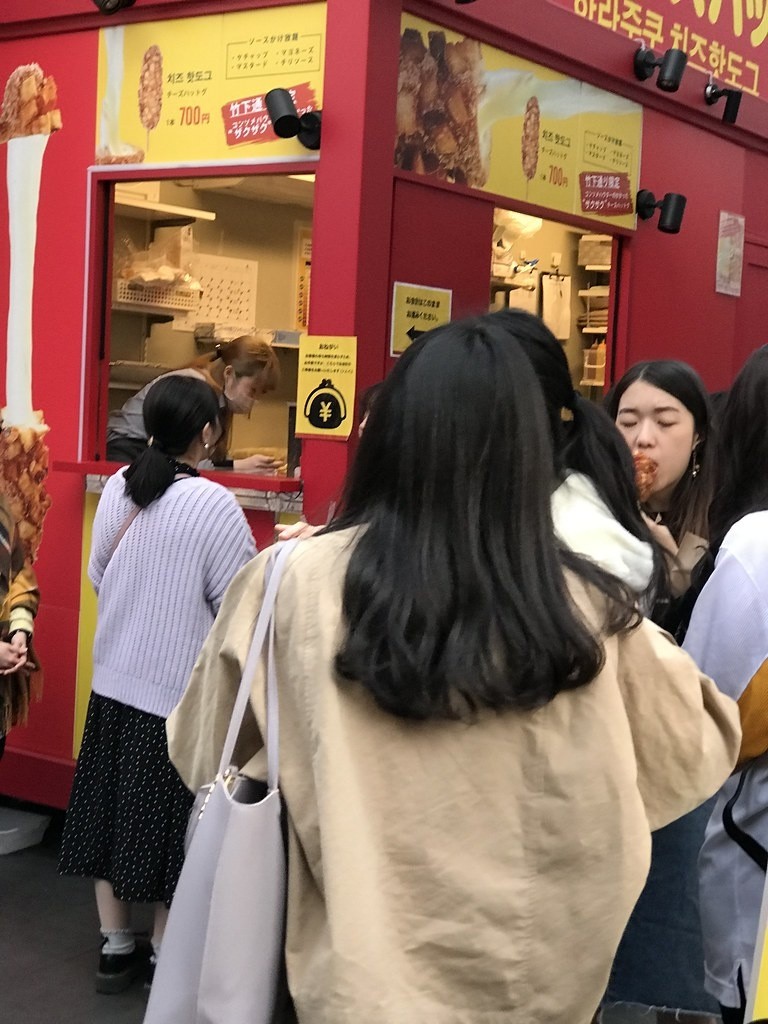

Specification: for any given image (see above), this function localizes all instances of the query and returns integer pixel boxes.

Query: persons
[54,376,259,996]
[162,317,768,1024]
[0,494,41,761]
[103,336,287,477]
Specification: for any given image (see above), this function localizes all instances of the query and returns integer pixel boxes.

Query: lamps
[636,189,687,234]
[704,83,743,123]
[93,0,136,15]
[265,88,322,149]
[633,46,687,93]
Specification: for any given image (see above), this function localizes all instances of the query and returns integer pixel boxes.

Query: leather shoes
[97,938,152,993]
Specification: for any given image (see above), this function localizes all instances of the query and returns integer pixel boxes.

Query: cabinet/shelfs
[108,196,216,390]
[578,234,613,385]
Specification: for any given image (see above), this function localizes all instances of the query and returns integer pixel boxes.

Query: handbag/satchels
[144,536,302,1024]
